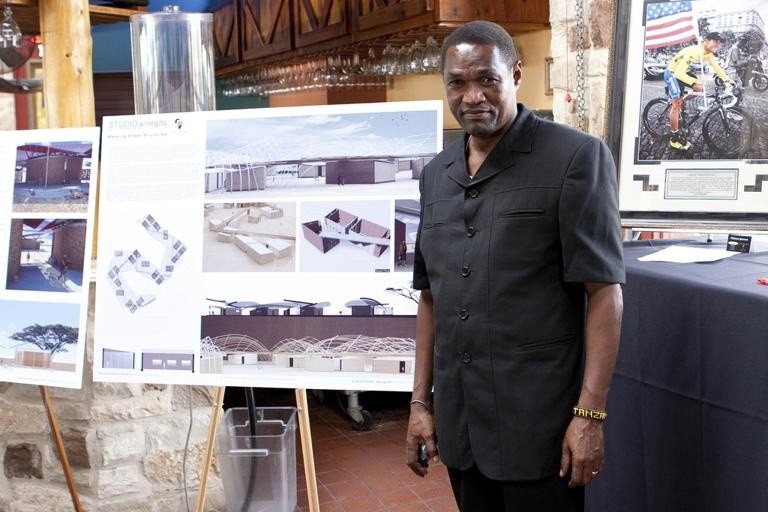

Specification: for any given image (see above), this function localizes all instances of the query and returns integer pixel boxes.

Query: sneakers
[671,130,687,146]
[726,112,743,121]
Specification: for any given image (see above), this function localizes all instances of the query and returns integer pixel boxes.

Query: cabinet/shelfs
[213,0,550,79]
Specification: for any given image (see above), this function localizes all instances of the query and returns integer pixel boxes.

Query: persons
[662,30,737,152]
[402,18,630,512]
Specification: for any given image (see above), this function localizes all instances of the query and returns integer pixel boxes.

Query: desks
[581,242,767,512]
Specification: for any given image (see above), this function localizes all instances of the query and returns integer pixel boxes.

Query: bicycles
[641,74,760,159]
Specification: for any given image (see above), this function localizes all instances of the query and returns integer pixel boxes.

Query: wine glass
[221,34,443,98]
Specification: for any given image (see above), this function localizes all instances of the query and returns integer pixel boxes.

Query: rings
[591,468,600,478]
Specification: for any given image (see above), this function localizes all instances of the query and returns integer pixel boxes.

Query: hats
[705,32,726,43]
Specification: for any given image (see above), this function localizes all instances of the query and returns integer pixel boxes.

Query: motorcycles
[645,34,767,94]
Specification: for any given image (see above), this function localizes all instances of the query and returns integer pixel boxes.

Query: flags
[643,0,698,52]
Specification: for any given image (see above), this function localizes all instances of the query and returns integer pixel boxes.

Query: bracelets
[572,404,609,425]
[409,398,430,412]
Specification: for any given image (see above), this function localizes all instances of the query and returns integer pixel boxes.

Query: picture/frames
[601,0,768,230]
